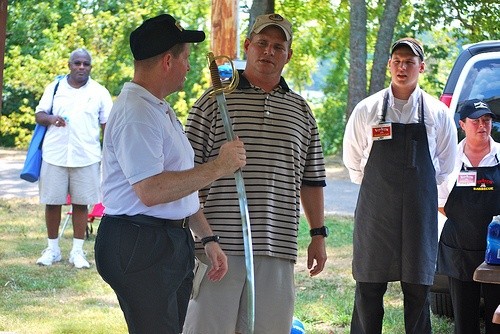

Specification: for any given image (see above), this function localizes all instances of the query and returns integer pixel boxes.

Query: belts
[108,213,189,228]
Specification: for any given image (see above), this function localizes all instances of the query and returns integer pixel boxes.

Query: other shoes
[68,250,90,269]
[35,246,62,265]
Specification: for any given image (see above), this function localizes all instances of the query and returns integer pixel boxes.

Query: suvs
[439,39,500,144]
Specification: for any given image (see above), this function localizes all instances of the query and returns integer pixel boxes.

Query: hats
[250,13,293,43]
[460,99,496,121]
[130,14,206,60]
[391,37,424,61]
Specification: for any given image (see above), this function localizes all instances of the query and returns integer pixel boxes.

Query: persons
[181,14,329,334]
[35,48,113,269]
[343,37,458,334]
[95,14,247,334]
[437,99,500,334]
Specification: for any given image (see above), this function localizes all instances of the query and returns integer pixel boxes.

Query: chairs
[59,195,104,240]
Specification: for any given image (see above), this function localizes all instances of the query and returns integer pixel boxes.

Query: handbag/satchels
[20,121,47,183]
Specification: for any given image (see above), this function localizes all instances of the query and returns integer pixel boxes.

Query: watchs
[310,226,329,237]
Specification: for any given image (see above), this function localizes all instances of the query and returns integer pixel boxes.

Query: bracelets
[201,235,219,246]
